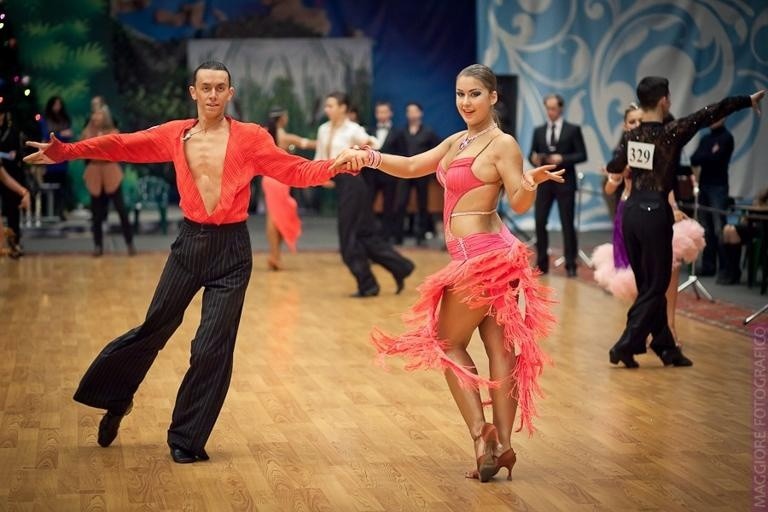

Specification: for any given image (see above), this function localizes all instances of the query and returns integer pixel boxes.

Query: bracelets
[521,175,537,192]
[364,144,382,168]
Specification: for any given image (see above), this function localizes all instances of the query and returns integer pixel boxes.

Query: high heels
[467,449,517,480]
[267,258,286,271]
[473,422,503,482]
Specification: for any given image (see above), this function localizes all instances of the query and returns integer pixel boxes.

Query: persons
[603,75,768,368]
[365,100,396,240]
[327,63,567,482]
[262,102,317,268]
[313,90,415,298]
[0,95,138,259]
[16,60,365,465]
[351,106,372,134]
[391,102,442,249]
[529,94,587,277]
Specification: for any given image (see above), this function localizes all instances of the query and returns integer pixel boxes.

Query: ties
[550,125,555,146]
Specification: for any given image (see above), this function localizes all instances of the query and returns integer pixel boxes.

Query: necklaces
[181,117,224,143]
[456,123,497,154]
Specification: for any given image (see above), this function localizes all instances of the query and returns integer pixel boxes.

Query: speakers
[494,73,518,138]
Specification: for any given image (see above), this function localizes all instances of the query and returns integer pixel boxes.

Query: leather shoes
[568,267,576,276]
[170,448,194,463]
[661,350,692,366]
[609,348,639,368]
[396,265,415,294]
[97,402,132,446]
[352,285,380,298]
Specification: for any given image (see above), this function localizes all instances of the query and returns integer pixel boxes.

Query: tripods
[552,172,591,269]
[674,183,713,302]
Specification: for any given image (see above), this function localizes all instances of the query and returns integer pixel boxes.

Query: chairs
[20,166,60,229]
